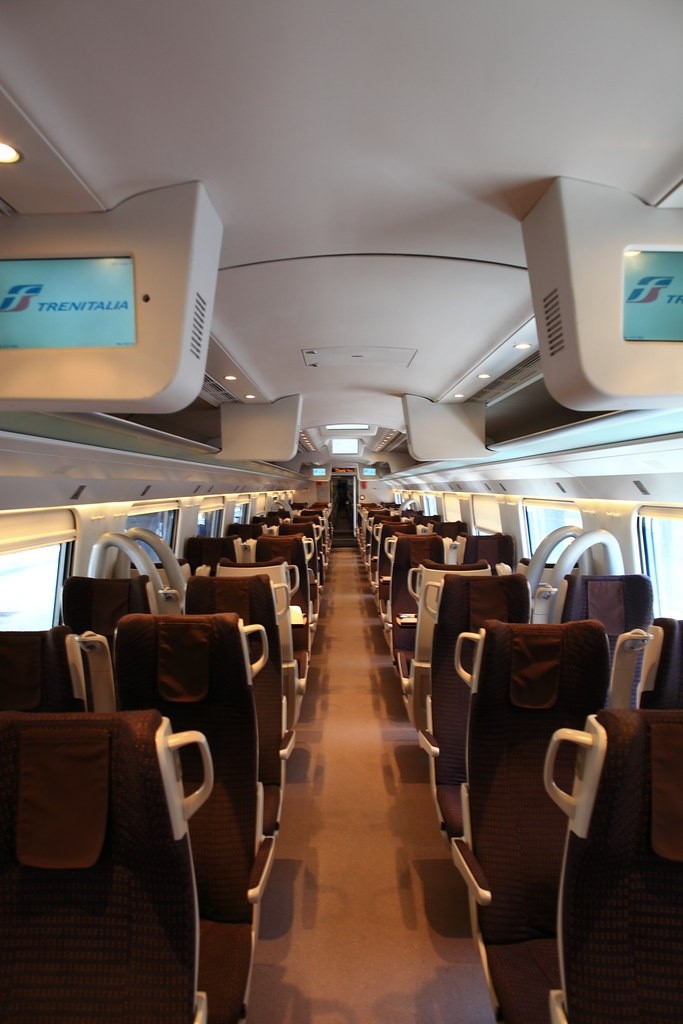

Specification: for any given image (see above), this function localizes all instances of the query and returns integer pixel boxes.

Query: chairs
[0,500,332,1024]
[354,499,683,1024]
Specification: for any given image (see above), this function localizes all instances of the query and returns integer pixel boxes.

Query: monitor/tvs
[1,253,134,350]
[622,242,683,342]
[363,467,376,476]
[312,467,326,476]
